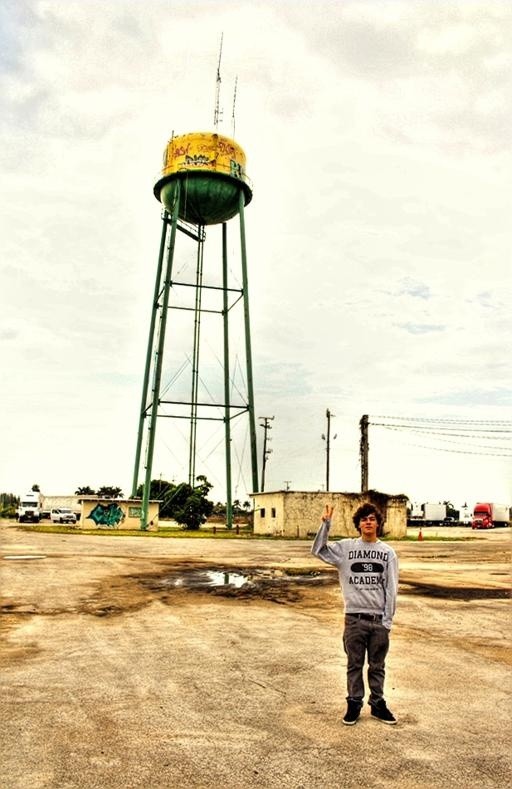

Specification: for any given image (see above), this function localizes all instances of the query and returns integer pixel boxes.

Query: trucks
[15,491,41,523]
[471,502,510,530]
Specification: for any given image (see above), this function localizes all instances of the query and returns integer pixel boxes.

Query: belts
[346,613,382,622]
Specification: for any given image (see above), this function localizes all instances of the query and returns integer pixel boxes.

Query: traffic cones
[416,527,425,540]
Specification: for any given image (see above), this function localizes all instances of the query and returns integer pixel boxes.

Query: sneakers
[343,700,363,724]
[371,699,397,725]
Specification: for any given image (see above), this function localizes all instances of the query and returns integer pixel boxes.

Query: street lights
[321,407,339,493]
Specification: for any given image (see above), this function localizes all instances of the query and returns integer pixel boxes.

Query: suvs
[50,507,77,524]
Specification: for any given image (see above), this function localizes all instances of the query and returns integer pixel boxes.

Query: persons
[310,501,398,726]
